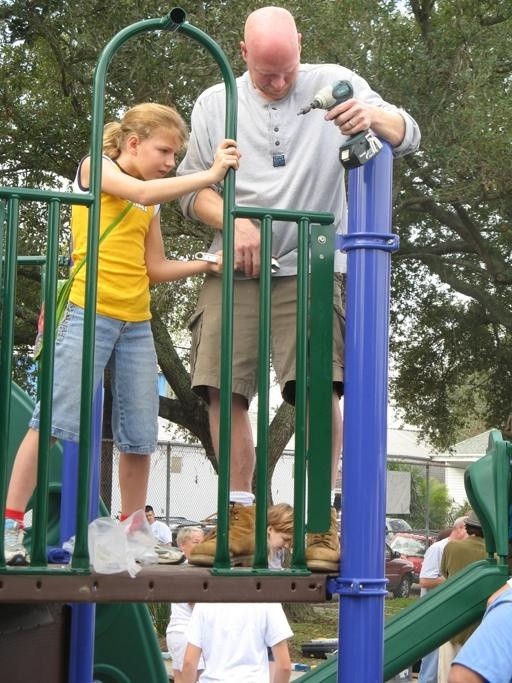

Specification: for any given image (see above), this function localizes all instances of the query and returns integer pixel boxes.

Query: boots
[306,508,341,573]
[189,502,256,567]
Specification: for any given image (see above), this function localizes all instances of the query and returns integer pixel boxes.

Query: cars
[385,517,413,534]
[384,541,414,600]
[385,530,440,585]
[156,511,217,547]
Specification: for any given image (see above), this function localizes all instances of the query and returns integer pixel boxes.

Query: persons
[1,101,241,564]
[173,3,423,574]
[145,505,294,682]
[418,512,512,683]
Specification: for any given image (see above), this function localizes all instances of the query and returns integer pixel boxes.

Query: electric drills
[297,80,384,170]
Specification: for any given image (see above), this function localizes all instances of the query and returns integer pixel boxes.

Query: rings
[348,119,355,128]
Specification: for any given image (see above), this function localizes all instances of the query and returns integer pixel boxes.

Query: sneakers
[4,519,29,564]
[153,544,186,565]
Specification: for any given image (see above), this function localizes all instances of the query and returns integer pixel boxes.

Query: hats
[463,511,481,527]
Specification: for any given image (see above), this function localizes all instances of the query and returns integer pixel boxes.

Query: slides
[288,561,506,682]
